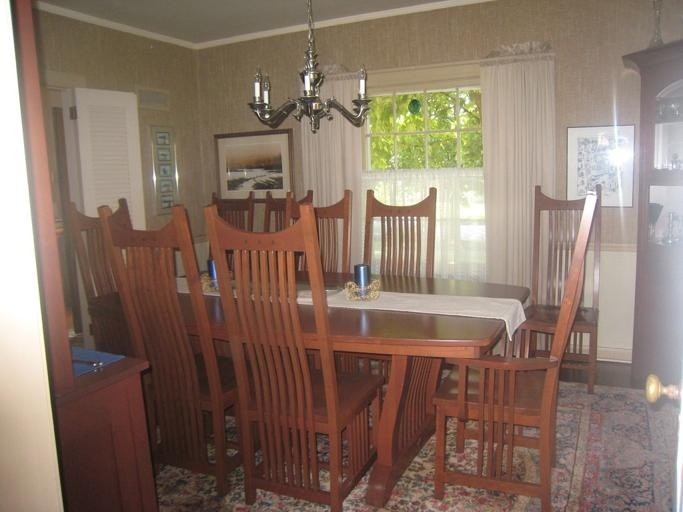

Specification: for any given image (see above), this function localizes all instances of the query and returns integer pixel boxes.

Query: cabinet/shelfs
[622,39,681,387]
[51,335,156,512]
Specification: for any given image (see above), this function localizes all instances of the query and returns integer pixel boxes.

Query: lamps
[246,1,376,131]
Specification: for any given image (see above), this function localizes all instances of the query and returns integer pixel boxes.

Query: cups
[353,264,370,300]
[207,256,225,292]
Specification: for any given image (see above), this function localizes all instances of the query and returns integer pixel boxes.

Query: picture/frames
[565,125,634,209]
[212,128,294,204]
[149,123,179,216]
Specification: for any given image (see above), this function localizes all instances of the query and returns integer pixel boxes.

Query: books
[73,346,125,379]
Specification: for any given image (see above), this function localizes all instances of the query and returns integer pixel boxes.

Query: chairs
[66,176,437,398]
[205,202,385,512]
[516,186,603,395]
[429,193,596,510]
[95,204,261,497]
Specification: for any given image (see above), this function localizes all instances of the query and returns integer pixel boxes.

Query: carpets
[150,356,676,510]
